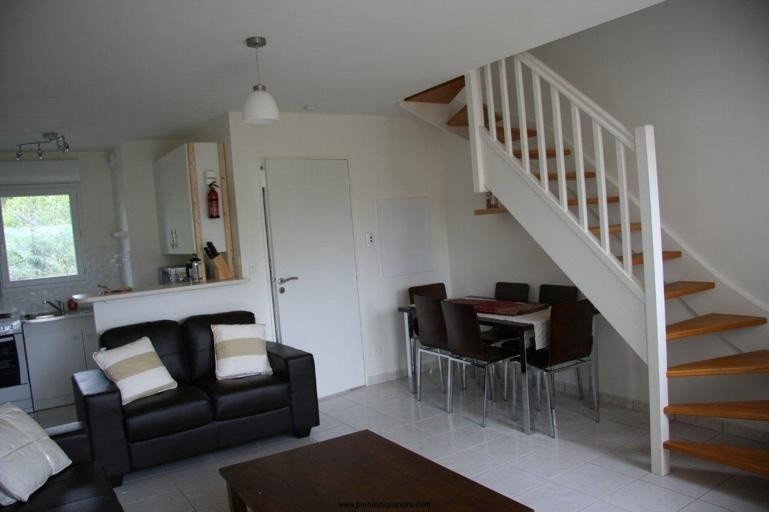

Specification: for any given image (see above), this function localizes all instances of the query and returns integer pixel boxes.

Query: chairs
[400,282,601,438]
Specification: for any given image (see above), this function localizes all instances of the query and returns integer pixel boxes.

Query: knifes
[204,242,219,259]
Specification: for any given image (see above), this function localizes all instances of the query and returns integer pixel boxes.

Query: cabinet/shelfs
[24,315,99,410]
[152,141,232,256]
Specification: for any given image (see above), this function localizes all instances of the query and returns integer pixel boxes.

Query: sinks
[25,311,59,319]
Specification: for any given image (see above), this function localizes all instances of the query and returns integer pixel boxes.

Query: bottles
[69,300,77,310]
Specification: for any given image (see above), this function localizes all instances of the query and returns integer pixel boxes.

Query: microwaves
[158,267,186,285]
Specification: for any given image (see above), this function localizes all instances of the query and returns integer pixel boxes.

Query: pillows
[94,335,178,407]
[1,402,75,503]
[0,491,16,508]
[210,323,273,382]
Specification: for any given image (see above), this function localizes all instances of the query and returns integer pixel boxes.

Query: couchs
[0,423,126,511]
[71,310,321,488]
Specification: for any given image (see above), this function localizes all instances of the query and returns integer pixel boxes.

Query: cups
[185,253,204,284]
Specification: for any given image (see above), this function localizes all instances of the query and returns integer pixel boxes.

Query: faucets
[43,299,66,315]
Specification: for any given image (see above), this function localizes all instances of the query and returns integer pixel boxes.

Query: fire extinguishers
[208,181,220,218]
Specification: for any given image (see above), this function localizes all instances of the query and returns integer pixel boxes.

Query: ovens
[0,333,34,413]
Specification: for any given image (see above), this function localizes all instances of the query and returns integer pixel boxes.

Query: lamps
[12,133,69,160]
[239,34,282,125]
